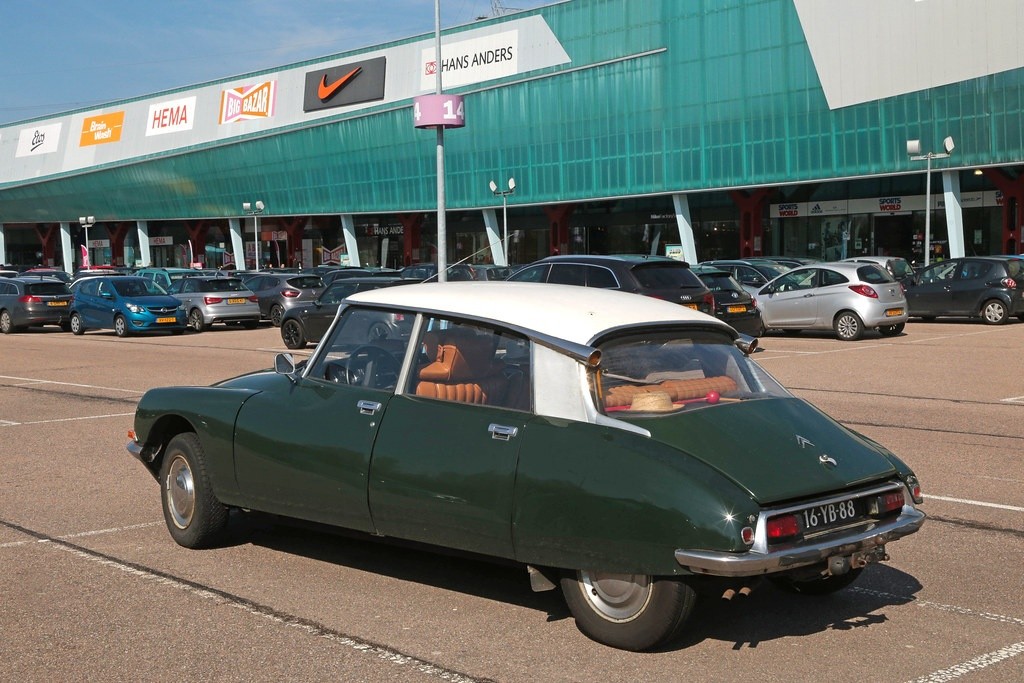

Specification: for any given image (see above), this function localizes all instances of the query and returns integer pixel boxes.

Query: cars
[0,264,528,328]
[128,280,927,653]
[798,256,914,290]
[900,255,1024,325]
[164,275,260,333]
[690,264,764,338]
[0,278,74,335]
[752,262,908,341]
[67,275,187,338]
[278,277,436,349]
[742,254,914,290]
[698,259,805,292]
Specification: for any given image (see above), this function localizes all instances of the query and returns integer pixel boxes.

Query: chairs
[415,328,508,406]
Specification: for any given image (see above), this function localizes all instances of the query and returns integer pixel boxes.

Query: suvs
[504,253,716,347]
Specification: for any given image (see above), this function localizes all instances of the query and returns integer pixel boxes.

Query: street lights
[489,177,516,263]
[242,200,265,272]
[78,216,96,271]
[906,135,955,277]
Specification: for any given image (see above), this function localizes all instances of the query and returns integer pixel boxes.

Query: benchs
[604,375,738,405]
